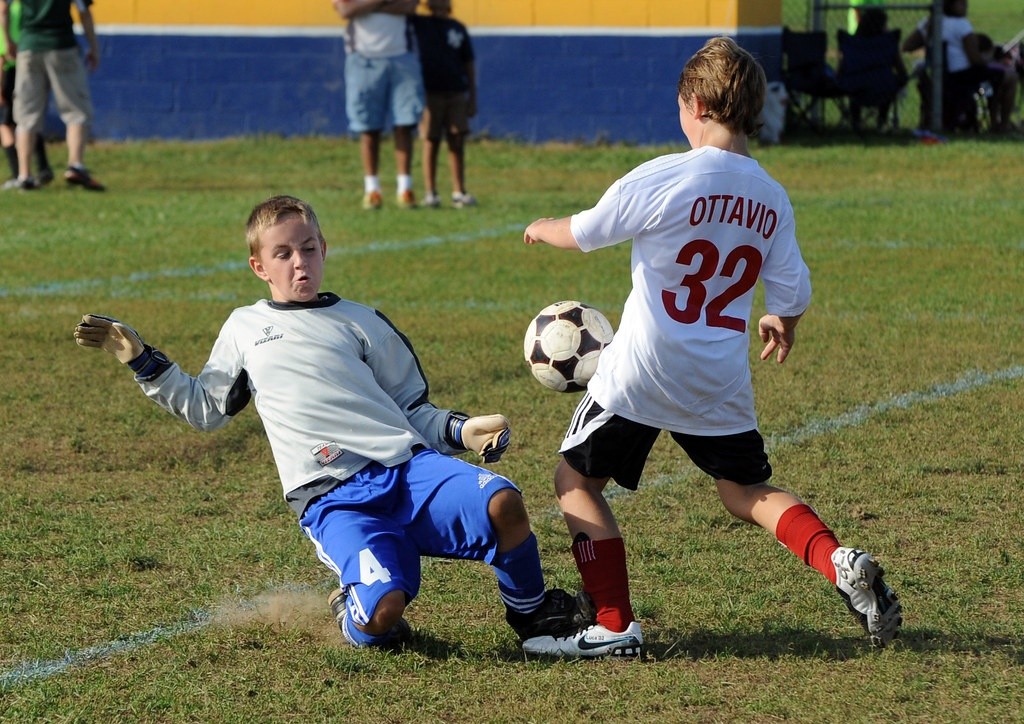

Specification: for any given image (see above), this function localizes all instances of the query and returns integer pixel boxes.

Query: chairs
[782,25,1024,144]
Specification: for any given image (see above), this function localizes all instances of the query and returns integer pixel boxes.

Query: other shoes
[35,168,54,187]
[3,175,18,188]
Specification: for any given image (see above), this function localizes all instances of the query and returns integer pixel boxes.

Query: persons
[842,9,904,132]
[522,38,903,658]
[333,0,479,211]
[902,0,974,132]
[74,196,595,655]
[0,0,106,193]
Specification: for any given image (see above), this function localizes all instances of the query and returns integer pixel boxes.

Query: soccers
[522,299,615,394]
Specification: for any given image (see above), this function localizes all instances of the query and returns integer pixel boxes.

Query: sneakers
[394,189,415,208]
[830,546,903,648]
[12,177,36,191]
[450,196,476,209]
[506,587,592,642]
[327,588,411,647]
[423,196,440,208]
[362,189,382,209]
[522,621,645,660]
[65,165,104,191]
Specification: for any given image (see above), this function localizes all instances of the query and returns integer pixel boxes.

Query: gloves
[73,313,171,381]
[445,411,512,464]
